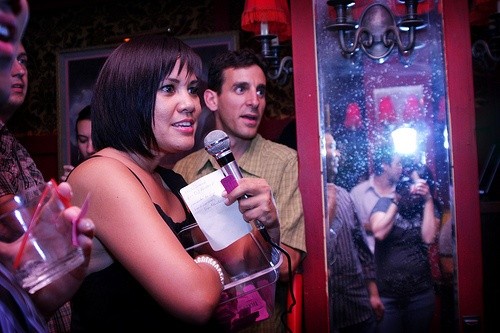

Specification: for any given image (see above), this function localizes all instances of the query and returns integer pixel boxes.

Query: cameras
[395,173,415,196]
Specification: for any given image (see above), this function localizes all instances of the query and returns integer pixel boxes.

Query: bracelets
[194,254,226,289]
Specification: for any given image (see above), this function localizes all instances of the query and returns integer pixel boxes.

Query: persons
[66,33,281,333]
[61,105,97,182]
[0,0,96,333]
[324,132,455,333]
[0,43,72,333]
[172,49,307,333]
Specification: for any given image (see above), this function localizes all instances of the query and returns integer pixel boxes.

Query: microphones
[203,130,271,242]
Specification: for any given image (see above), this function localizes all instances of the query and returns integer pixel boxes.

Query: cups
[0,182,84,295]
